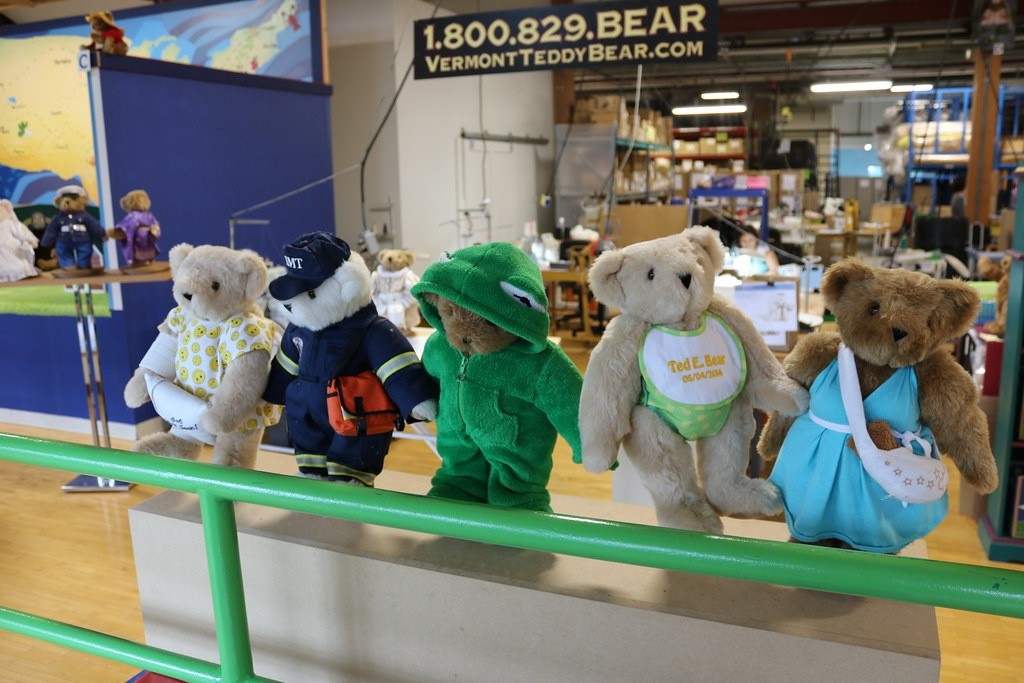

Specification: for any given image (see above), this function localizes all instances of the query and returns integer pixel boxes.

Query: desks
[841,223,891,260]
[0,261,172,493]
[540,266,601,345]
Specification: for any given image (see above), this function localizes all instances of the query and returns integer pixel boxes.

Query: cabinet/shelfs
[651,126,750,161]
[551,121,675,239]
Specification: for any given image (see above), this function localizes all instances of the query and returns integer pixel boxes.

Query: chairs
[555,241,612,338]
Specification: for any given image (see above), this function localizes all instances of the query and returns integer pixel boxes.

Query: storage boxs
[573,96,744,195]
[814,203,909,266]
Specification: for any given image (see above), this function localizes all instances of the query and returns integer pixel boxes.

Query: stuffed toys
[261,232,441,491]
[108,190,161,267]
[123,243,284,470]
[759,258,998,555]
[981,247,1011,337]
[41,186,107,271]
[577,225,810,536]
[407,242,618,515]
[368,248,423,337]
[81,11,127,55]
[0,199,39,283]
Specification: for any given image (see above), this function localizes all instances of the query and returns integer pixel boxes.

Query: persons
[736,226,780,272]
